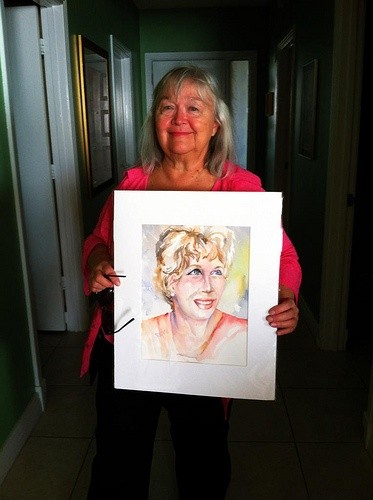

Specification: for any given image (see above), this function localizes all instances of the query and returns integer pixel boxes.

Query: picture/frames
[112,188,283,403]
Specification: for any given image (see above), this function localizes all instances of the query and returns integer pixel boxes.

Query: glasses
[93,272,135,336]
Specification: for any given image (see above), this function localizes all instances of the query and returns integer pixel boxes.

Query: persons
[77,64,302,500]
